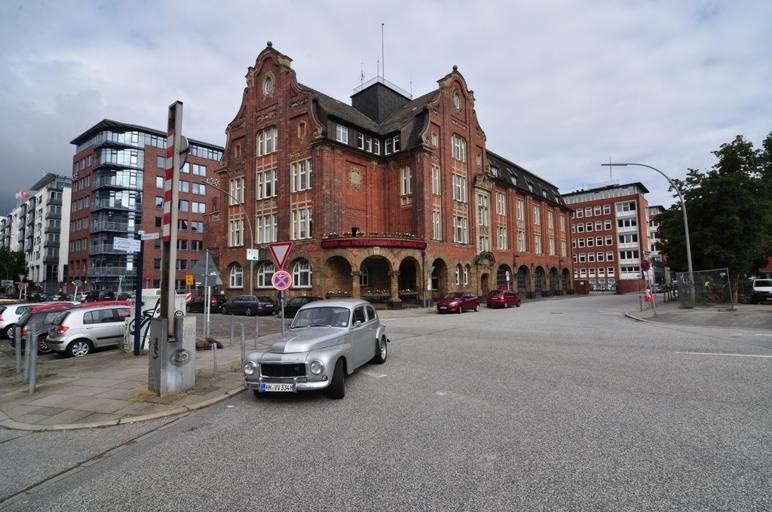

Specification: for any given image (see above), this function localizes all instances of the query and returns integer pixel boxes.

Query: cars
[187,293,324,318]
[243,298,390,399]
[750,278,772,304]
[0,299,133,358]
[486,289,521,309]
[28,288,177,304]
[436,289,480,314]
[650,280,680,293]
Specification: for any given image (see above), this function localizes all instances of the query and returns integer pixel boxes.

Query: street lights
[200,179,254,295]
[601,163,696,299]
